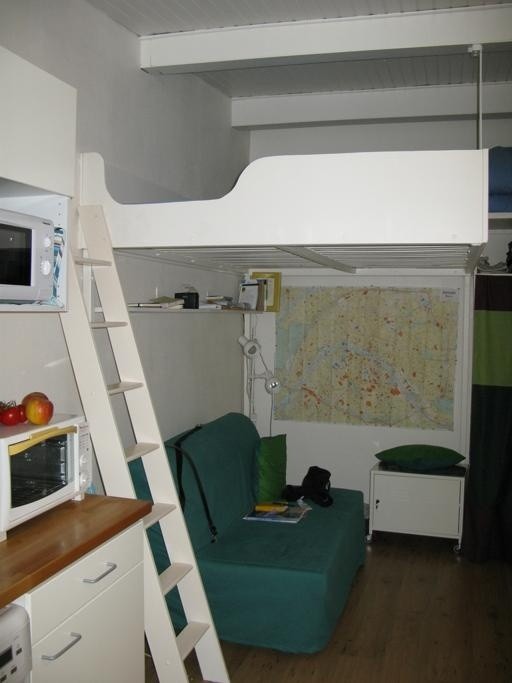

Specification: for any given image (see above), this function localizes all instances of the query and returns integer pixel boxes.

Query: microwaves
[0,207,57,302]
[0,411,95,542]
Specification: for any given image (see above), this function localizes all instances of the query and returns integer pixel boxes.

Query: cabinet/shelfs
[364,464,467,552]
[12,517,146,683]
[0,45,78,314]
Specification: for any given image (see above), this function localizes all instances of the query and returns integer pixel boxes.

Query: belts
[175,424,204,513]
[165,445,218,543]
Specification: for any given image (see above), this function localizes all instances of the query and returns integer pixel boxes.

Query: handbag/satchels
[286,466,333,508]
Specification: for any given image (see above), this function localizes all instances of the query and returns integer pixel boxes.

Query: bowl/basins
[174,291,199,310]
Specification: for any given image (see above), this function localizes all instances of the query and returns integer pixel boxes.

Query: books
[125,270,282,311]
[241,499,311,523]
[478,261,509,274]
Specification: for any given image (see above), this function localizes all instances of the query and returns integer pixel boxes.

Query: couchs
[129,411,367,655]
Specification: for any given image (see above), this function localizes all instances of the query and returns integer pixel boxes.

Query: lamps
[238,314,282,419]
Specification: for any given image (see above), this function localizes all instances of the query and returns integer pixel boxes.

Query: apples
[22,391,53,425]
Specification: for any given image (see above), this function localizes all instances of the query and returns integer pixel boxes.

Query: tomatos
[0,401,26,426]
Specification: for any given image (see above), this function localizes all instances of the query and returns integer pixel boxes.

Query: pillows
[253,433,290,504]
[373,441,466,471]
